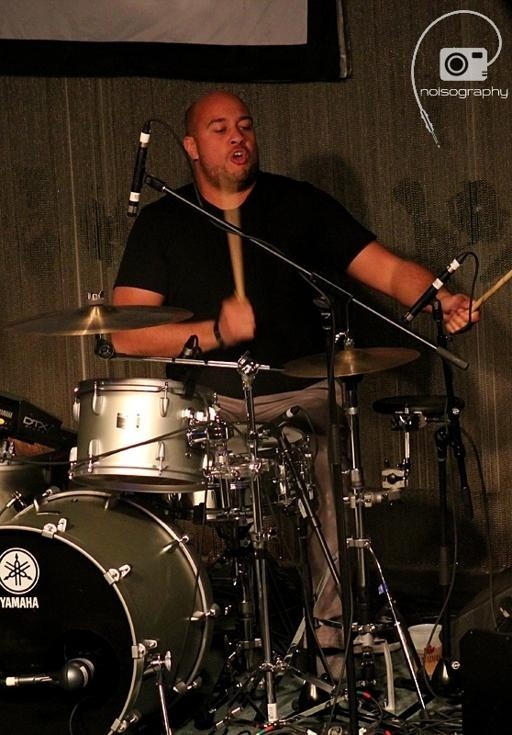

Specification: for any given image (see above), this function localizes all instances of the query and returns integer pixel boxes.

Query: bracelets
[214,317,224,350]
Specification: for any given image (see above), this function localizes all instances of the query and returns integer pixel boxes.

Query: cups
[407,625,449,681]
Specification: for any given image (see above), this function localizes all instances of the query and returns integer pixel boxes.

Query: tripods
[95,173,472,735]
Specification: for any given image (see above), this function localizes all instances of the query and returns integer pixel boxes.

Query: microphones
[127,130,150,218]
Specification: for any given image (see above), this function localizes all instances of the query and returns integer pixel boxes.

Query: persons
[109,89,480,683]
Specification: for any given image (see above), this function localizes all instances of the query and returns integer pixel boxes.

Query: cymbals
[279,346,421,378]
[1,306,194,337]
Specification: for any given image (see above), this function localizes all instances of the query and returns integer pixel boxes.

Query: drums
[0,491,214,735]
[72,378,203,495]
